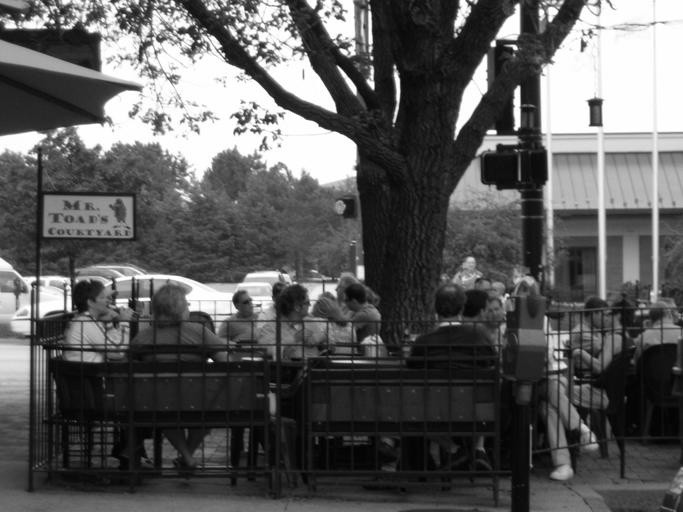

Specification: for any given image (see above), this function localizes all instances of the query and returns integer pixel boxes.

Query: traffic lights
[334,195,355,220]
[530,146,547,187]
[485,45,515,134]
[479,146,519,186]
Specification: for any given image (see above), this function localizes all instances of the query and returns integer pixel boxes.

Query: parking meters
[498,275,545,511]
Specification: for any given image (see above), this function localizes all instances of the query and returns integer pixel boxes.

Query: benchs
[49,344,535,480]
[635,344,680,444]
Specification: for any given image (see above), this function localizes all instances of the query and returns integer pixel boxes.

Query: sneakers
[549,466,575,482]
[578,432,599,452]
[419,458,435,483]
[139,456,155,471]
[172,455,199,471]
[473,451,494,481]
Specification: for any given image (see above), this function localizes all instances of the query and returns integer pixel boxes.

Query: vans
[244,269,290,291]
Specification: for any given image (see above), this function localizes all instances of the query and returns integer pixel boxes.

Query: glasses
[297,300,312,307]
[342,298,354,303]
[606,312,617,317]
[184,301,191,307]
[237,298,253,306]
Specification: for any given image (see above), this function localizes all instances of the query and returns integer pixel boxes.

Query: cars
[283,272,298,287]
[232,281,274,311]
[11,272,271,340]
[0,256,146,337]
